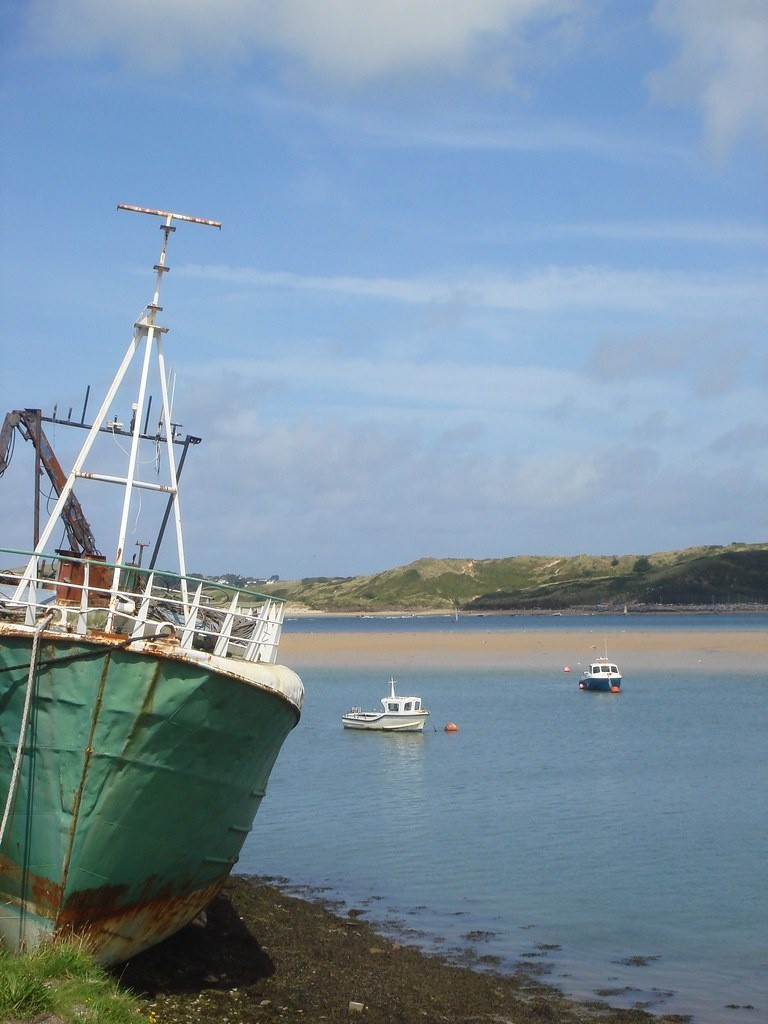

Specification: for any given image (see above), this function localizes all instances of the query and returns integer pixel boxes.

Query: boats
[0,203,307,977]
[577,639,622,692]
[340,678,431,733]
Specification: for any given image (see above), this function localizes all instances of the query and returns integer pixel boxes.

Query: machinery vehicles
[0,405,113,609]
